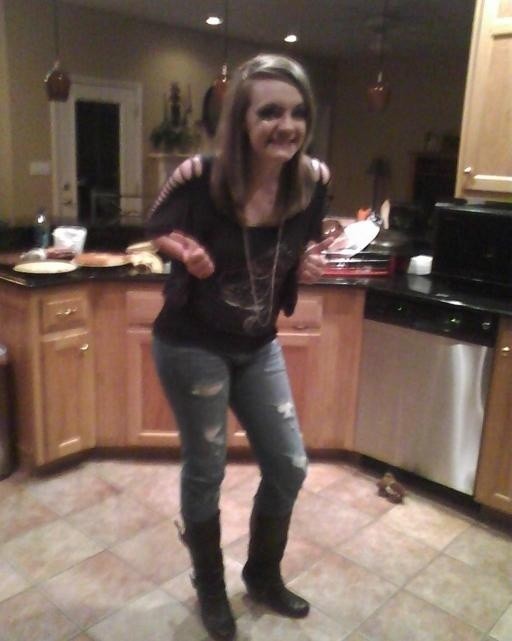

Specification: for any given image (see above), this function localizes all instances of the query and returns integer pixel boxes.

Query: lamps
[44,1,71,104]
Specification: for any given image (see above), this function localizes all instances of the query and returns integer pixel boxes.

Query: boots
[174,509,236,641]
[242,511,310,618]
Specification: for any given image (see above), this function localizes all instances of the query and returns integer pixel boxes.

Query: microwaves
[428,202,512,300]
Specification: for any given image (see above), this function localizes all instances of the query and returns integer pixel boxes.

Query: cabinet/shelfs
[95,280,365,459]
[472,321,511,521]
[453,1,511,203]
[1,282,97,465]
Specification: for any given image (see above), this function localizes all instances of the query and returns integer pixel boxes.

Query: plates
[72,253,132,268]
[13,262,78,274]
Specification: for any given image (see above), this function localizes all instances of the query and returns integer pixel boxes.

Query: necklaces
[237,204,287,329]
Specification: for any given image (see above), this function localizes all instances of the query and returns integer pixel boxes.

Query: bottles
[28,207,52,249]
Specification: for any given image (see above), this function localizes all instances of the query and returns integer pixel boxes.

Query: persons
[147,56,334,641]
